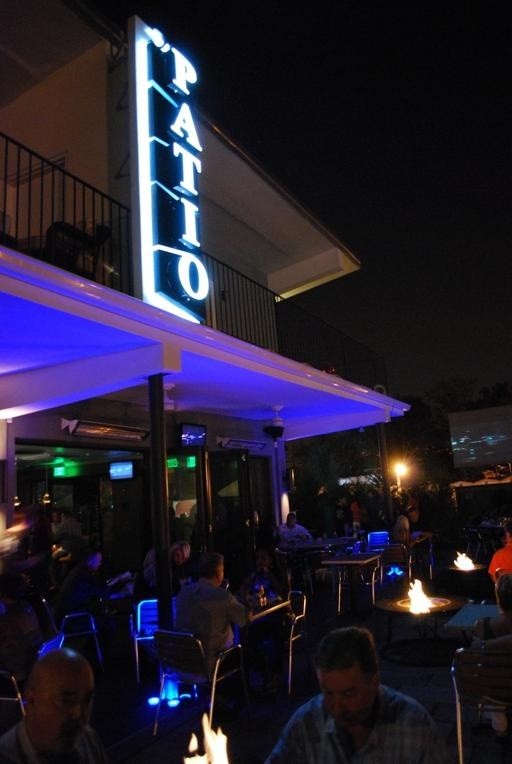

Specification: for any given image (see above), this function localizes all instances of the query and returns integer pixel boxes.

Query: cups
[303,535,312,545]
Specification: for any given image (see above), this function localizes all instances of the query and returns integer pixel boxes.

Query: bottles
[257,583,272,608]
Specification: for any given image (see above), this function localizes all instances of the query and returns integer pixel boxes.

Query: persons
[2,482,511,764]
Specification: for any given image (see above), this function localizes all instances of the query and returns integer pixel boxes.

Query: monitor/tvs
[180,422,207,449]
[108,460,135,481]
